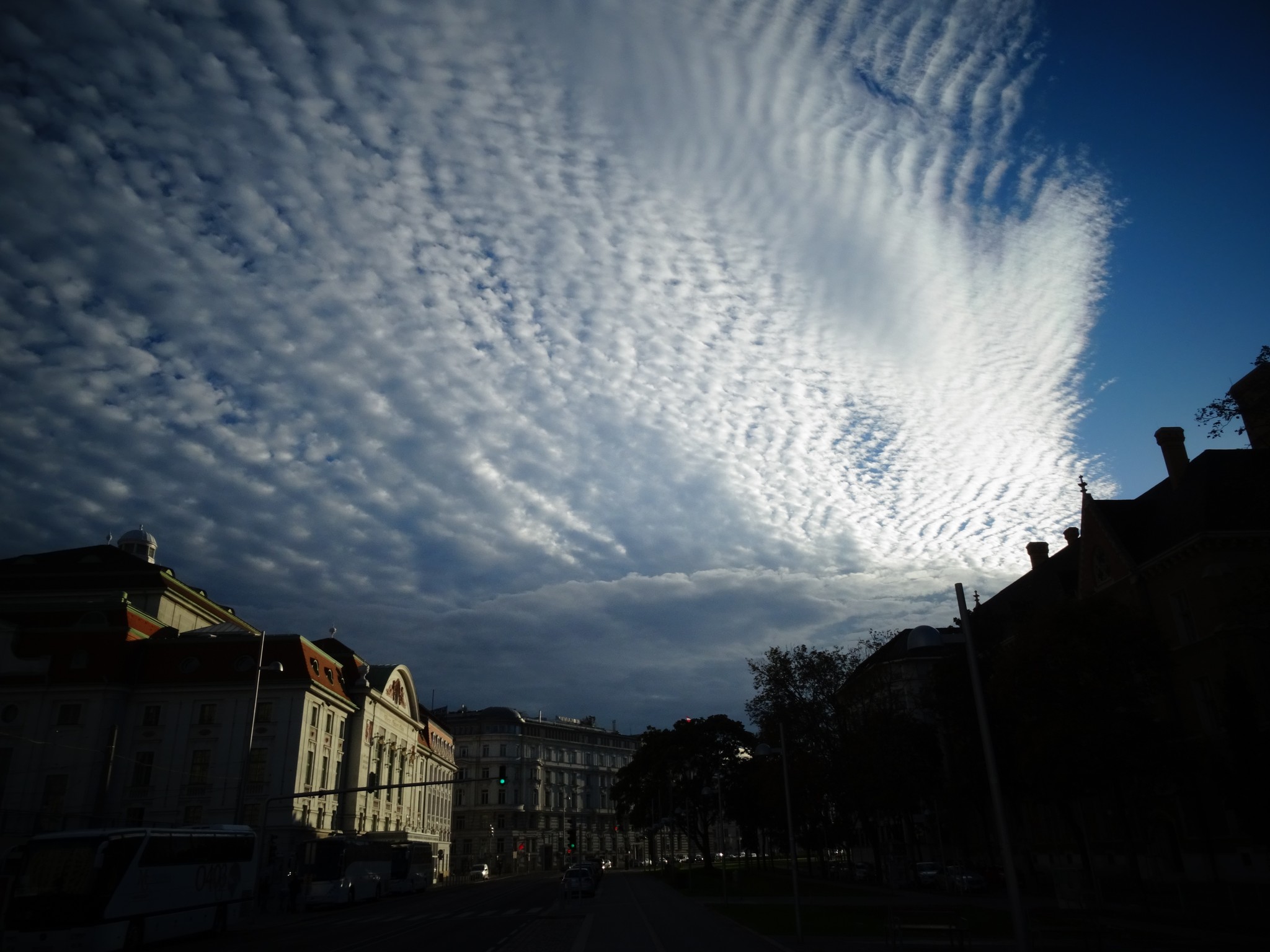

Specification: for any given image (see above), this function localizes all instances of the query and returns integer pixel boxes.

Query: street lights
[233,630,283,824]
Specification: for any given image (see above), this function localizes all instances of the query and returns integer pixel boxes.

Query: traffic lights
[567,829,576,849]
[499,765,506,786]
[568,849,571,856]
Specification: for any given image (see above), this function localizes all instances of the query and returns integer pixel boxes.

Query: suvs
[675,855,689,863]
[634,858,652,868]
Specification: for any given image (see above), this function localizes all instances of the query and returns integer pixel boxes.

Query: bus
[297,831,435,910]
[0,825,260,952]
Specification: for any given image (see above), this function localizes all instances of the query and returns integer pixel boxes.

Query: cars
[560,857,612,899]
[653,857,668,866]
[713,852,757,862]
[693,855,704,863]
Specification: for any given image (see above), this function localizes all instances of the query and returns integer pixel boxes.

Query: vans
[469,864,489,881]
